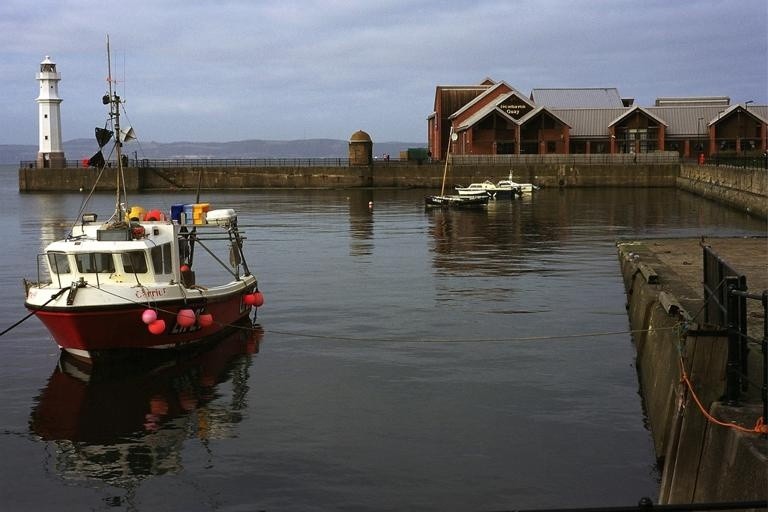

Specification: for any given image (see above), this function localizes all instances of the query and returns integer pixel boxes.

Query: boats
[31,317,261,484]
[25,38,263,357]
[425,179,533,205]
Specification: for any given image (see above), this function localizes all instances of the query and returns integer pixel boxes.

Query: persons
[383,153,387,161]
[388,154,390,160]
[177,226,189,264]
[428,151,432,165]
